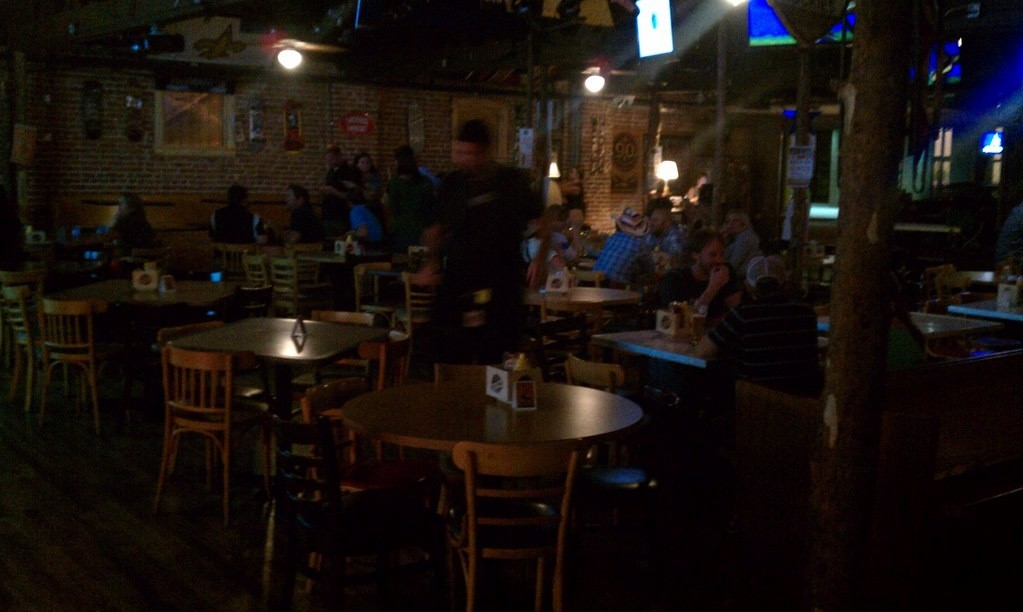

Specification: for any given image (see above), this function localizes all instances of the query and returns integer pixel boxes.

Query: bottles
[997,266,1010,311]
[526,367,547,409]
[512,353,530,382]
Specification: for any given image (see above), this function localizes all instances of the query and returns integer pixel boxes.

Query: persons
[284,184,327,245]
[522,204,580,271]
[592,207,657,290]
[696,258,819,396]
[381,145,435,275]
[319,144,388,262]
[640,196,689,288]
[208,184,269,244]
[410,120,550,363]
[113,193,155,261]
[719,208,787,287]
[995,202,1023,263]
[656,228,742,315]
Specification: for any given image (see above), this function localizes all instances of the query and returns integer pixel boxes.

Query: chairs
[0,227,1023,612]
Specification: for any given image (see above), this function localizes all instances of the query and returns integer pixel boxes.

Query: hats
[746,257,785,288]
[618,207,650,237]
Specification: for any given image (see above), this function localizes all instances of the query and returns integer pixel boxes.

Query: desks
[71,277,243,347]
[166,315,391,426]
[522,285,643,359]
[947,299,1023,323]
[591,329,724,396]
[338,377,645,524]
[908,310,1006,345]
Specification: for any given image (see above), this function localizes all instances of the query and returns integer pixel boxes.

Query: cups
[688,301,709,354]
